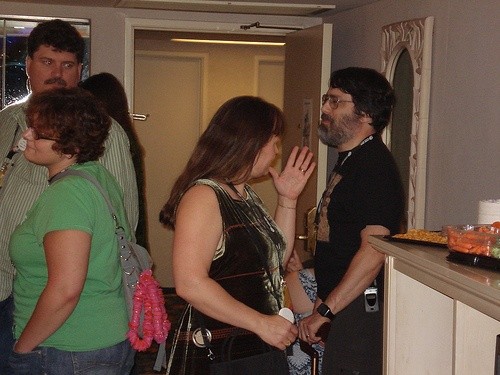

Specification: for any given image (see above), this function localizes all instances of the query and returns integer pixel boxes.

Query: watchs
[318,303,336,321]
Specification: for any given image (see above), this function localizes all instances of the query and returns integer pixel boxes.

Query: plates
[383,231,448,247]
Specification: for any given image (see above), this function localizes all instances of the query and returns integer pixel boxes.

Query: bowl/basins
[442,224,500,258]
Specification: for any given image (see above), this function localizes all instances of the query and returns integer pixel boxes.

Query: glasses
[24,114,61,143]
[321,93,353,110]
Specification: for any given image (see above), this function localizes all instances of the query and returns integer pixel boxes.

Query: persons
[160,95,316,375]
[0,19,150,375]
[298,67,404,375]
[283,206,326,375]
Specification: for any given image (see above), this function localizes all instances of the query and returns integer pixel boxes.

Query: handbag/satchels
[115,224,160,339]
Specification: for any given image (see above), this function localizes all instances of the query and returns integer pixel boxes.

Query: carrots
[447,221,500,256]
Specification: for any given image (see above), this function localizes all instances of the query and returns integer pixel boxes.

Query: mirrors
[382,16,435,229]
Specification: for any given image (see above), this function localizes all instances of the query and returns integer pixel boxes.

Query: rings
[306,334,310,338]
[300,168,306,172]
[285,340,291,345]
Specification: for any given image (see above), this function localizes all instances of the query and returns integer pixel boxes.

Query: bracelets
[278,204,297,210]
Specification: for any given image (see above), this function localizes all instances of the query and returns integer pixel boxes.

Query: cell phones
[364,279,379,312]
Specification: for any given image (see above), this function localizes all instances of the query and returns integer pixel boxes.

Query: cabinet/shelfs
[382,253,500,375]
